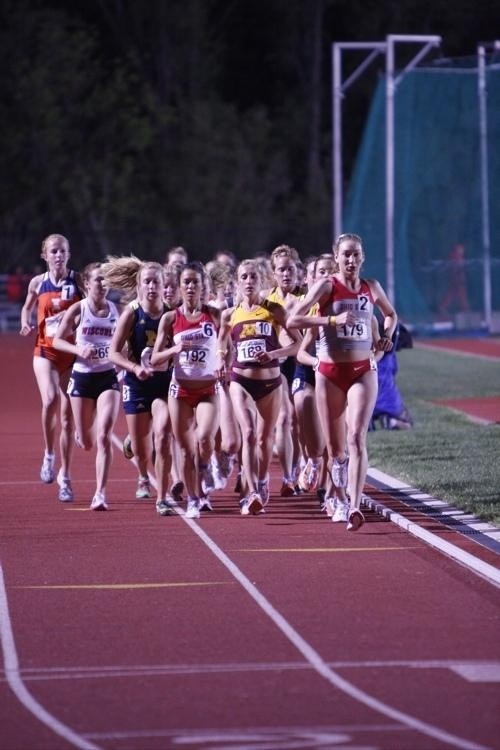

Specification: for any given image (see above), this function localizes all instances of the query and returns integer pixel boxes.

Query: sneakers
[331,457,348,488]
[185,496,202,519]
[56,469,74,502]
[199,449,271,516]
[90,491,108,511]
[368,413,390,432]
[345,507,365,532]
[155,499,172,516]
[317,487,350,522]
[122,433,134,459]
[40,448,56,484]
[135,475,152,499]
[276,454,324,497]
[170,479,184,501]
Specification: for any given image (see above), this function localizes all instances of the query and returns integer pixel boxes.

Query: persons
[436,243,473,319]
[6,224,401,533]
